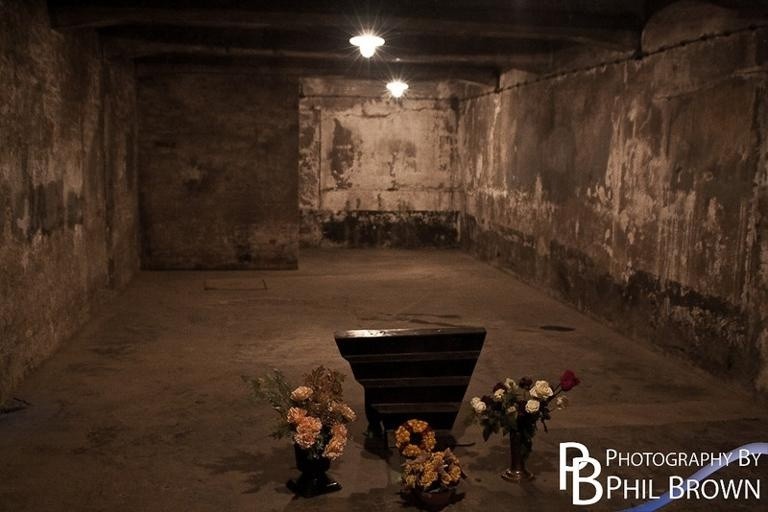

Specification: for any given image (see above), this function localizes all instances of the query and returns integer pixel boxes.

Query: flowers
[395,414,472,501]
[243,362,357,467]
[469,369,581,451]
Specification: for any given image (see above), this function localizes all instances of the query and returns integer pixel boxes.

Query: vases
[500,431,534,483]
[420,487,451,508]
[284,441,342,499]
